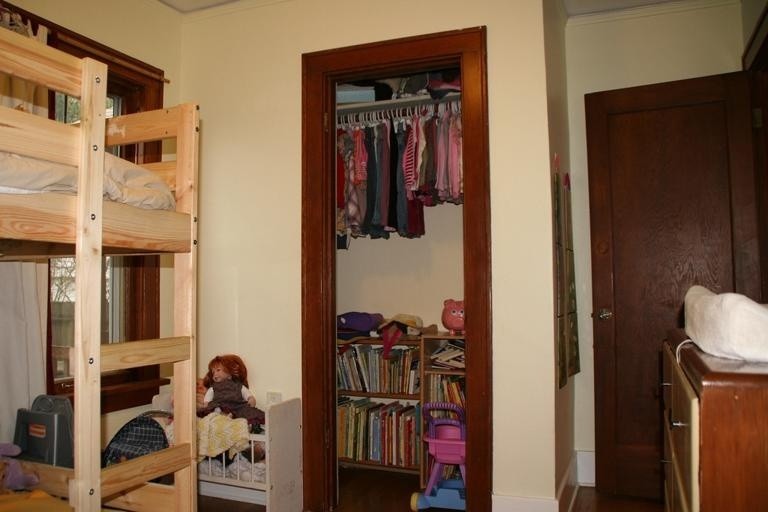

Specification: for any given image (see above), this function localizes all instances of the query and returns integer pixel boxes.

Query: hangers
[335,101,465,128]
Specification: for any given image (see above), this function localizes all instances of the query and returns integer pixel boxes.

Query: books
[333,333,464,476]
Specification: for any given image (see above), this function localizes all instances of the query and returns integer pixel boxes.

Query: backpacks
[102,411,173,485]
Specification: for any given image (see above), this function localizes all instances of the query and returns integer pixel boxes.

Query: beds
[151,381,305,510]
[0,26,199,512]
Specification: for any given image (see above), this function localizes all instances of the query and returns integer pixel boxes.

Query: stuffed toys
[439,296,468,335]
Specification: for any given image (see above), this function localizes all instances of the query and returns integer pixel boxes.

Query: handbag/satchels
[684,284,768,362]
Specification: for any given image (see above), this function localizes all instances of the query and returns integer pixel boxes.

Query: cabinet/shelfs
[334,334,465,488]
[660,335,767,510]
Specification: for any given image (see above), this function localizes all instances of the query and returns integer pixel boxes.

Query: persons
[198,352,265,463]
